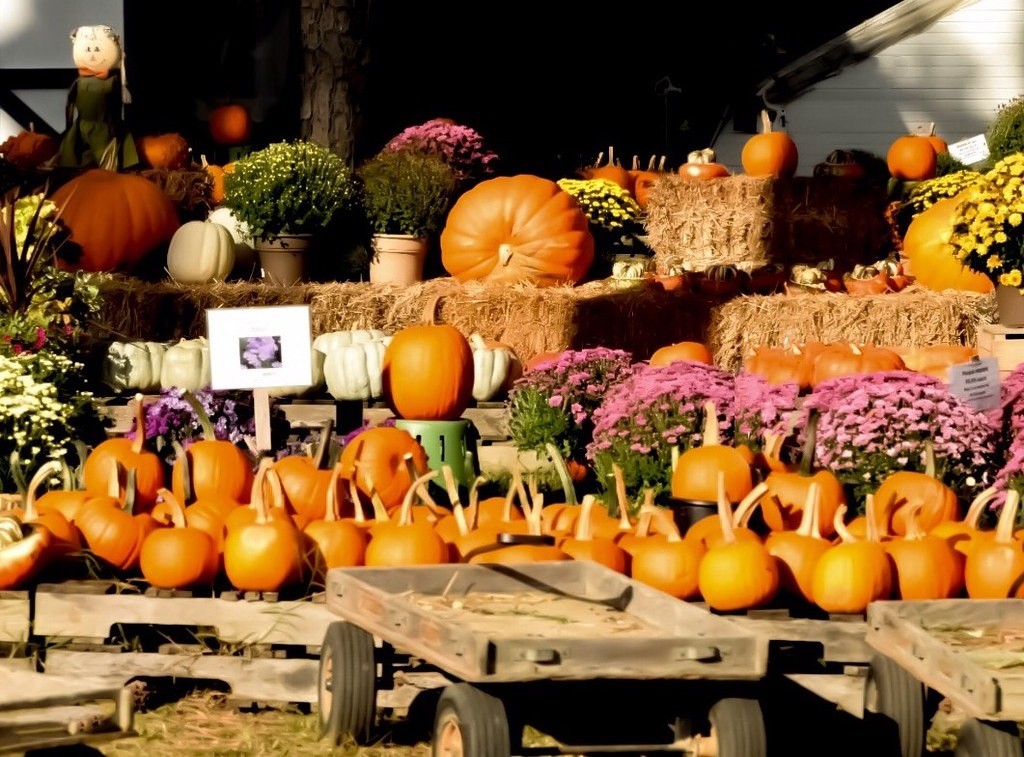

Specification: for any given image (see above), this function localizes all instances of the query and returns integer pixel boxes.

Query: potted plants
[785,258,841,297]
[353,142,459,290]
[841,264,887,296]
[873,258,911,294]
[610,254,784,299]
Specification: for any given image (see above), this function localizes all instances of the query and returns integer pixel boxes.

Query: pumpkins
[0,105,1024,613]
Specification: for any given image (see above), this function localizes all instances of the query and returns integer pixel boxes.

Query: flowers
[0,173,115,498]
[885,93,1024,300]
[217,136,356,247]
[122,382,257,451]
[382,117,499,183]
[501,345,1024,528]
[555,176,643,237]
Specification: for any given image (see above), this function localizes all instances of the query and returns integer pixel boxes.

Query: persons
[45,24,141,172]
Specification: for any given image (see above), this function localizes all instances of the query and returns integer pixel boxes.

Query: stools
[388,417,482,502]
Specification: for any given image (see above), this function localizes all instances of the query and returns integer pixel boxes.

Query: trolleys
[862,597,1024,757]
[316,557,770,757]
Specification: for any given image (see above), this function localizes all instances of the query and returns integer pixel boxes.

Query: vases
[560,453,594,488]
[667,496,744,539]
[250,232,315,286]
[994,282,1024,329]
